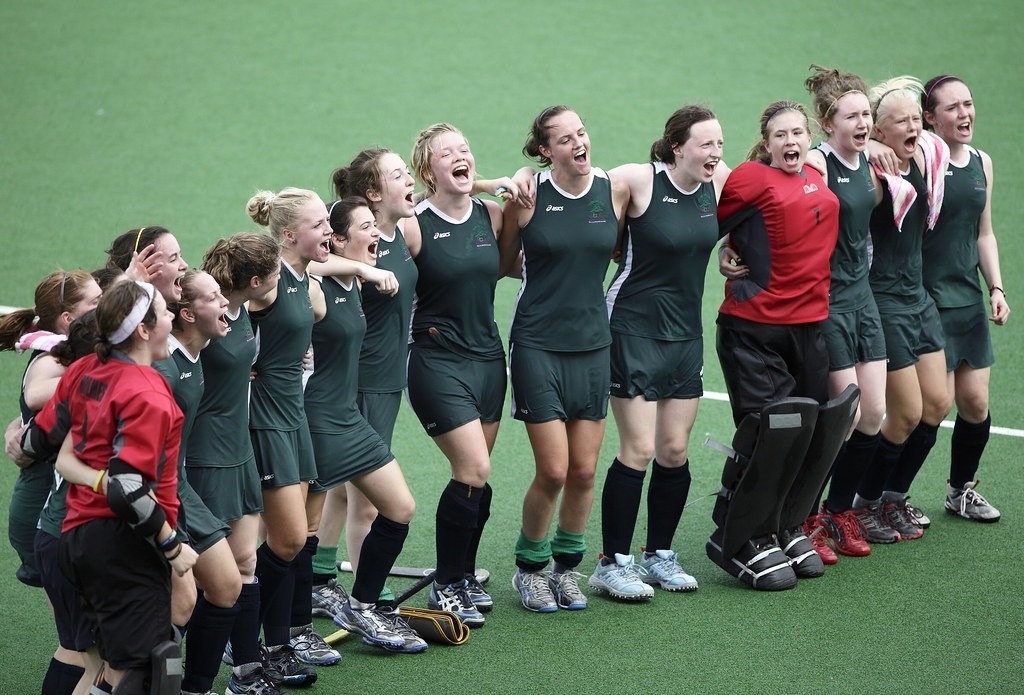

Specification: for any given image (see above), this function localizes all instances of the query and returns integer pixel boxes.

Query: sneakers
[363,600,429,652]
[221,638,284,687]
[334,598,406,648]
[802,514,838,564]
[311,577,348,618]
[512,567,559,612]
[945,479,1001,522]
[289,623,342,666]
[587,553,654,600]
[638,546,699,592]
[817,499,870,556]
[265,642,318,687]
[852,499,901,543]
[225,663,289,695]
[428,579,485,629]
[548,570,588,609]
[905,501,931,529]
[879,496,924,540]
[462,573,494,612]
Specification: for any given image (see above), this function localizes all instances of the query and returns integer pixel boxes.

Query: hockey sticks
[323,569,437,642]
[337,560,491,585]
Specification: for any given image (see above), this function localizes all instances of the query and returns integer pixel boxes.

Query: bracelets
[98,478,104,496]
[159,528,176,546]
[165,540,182,560]
[717,242,731,252]
[93,470,105,497]
[160,534,179,552]
[989,285,1006,297]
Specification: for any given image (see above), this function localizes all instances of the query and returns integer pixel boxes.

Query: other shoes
[706,526,798,590]
[783,526,825,578]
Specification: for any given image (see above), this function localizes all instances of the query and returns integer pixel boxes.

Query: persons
[864,76,1011,528]
[0,63,952,695]
